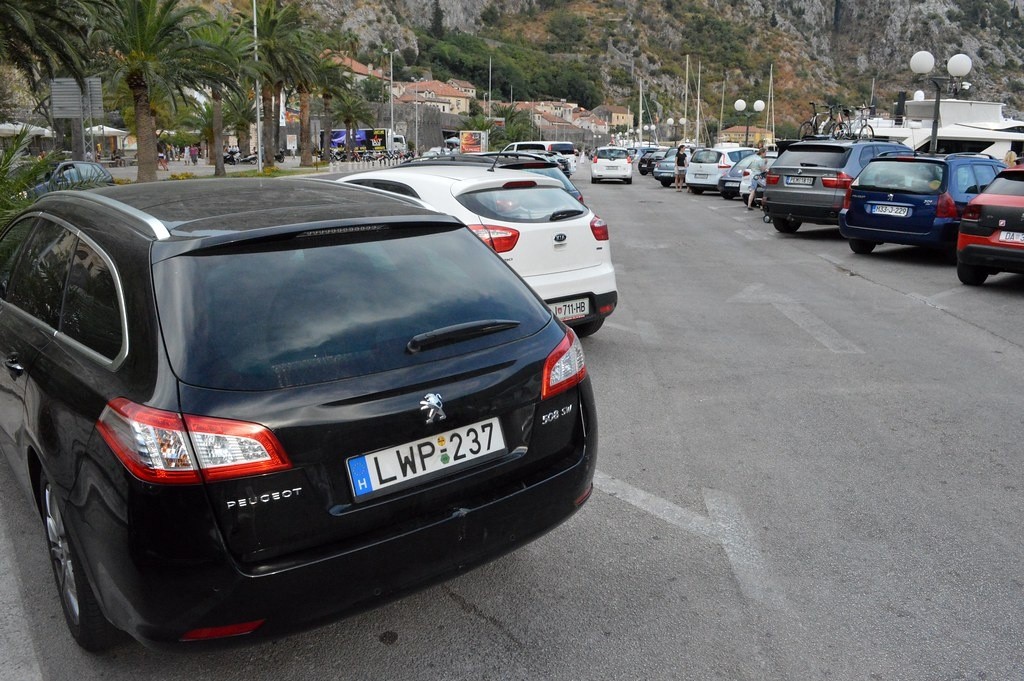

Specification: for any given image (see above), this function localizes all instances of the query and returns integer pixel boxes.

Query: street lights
[734,98,766,148]
[667,116,687,148]
[908,49,975,155]
[592,124,657,148]
[382,45,400,158]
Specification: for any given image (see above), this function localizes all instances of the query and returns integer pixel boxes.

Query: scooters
[224,145,286,164]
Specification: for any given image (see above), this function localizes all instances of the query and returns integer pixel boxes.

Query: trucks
[318,126,408,157]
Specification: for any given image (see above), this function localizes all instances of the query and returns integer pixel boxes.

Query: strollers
[754,169,774,223]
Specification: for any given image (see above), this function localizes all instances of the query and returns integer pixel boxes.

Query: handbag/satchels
[754,170,766,187]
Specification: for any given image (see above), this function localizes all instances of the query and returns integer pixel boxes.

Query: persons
[261,145,264,159]
[37,150,46,161]
[747,148,769,210]
[157,143,199,171]
[291,146,295,160]
[1002,151,1017,166]
[675,145,687,192]
[223,144,239,153]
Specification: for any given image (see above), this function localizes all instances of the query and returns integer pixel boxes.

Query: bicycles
[313,147,410,161]
[795,98,878,140]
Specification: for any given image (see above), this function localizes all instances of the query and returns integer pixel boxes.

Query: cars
[0,178,601,661]
[638,137,1024,286]
[328,141,619,341]
[1,160,115,206]
[591,146,635,185]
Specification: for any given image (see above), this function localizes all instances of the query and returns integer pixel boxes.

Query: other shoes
[747,206,753,210]
[676,187,681,192]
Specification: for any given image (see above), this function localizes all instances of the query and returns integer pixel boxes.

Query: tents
[86,124,129,141]
[0,121,65,149]
[444,137,459,148]
